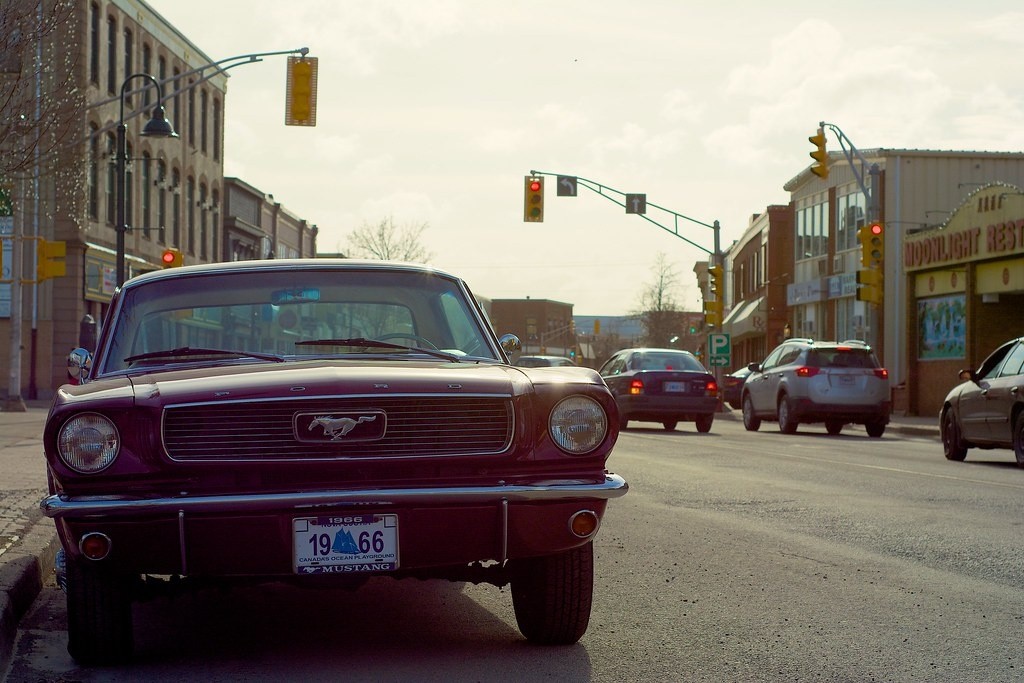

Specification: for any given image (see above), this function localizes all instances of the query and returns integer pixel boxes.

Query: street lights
[112,75,179,291]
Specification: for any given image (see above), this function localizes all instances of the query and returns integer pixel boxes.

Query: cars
[514,355,577,370]
[594,347,719,437]
[937,336,1024,466]
[40,256,632,669]
[721,359,763,410]
[739,338,892,438]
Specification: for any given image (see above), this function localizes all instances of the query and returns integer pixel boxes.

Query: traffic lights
[855,266,882,304]
[857,227,870,267]
[38,240,66,279]
[161,247,183,270]
[808,129,828,181]
[707,266,722,297]
[525,175,544,223]
[868,222,884,260]
[704,301,721,326]
